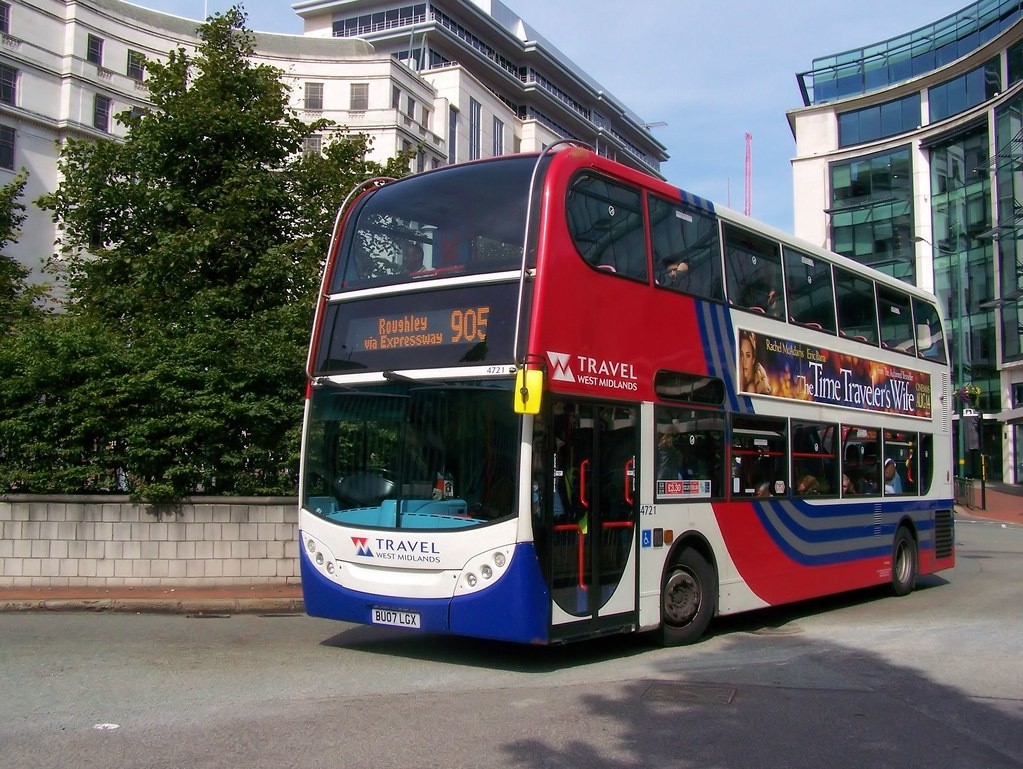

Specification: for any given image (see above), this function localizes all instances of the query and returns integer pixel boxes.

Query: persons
[398,245,428,282]
[640,253,791,315]
[398,398,920,531]
[738,330,770,393]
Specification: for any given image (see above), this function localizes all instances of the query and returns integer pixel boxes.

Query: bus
[296,140,959,651]
